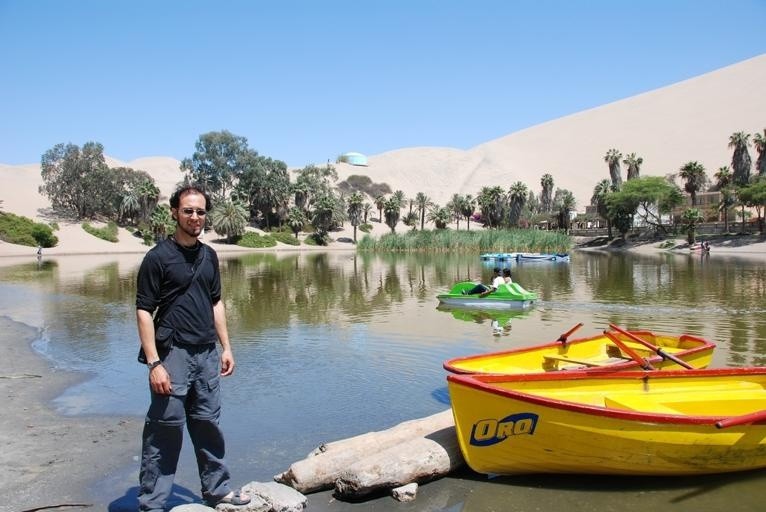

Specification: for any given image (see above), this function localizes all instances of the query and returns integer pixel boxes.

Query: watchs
[147,360,160,370]
[135,187,252,511]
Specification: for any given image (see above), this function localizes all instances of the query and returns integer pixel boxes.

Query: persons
[461,267,505,298]
[502,268,513,284]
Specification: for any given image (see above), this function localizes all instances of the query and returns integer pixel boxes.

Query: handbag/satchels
[137,327,174,365]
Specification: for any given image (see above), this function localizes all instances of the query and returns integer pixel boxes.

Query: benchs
[605,395,683,415]
[607,341,686,354]
[542,354,601,367]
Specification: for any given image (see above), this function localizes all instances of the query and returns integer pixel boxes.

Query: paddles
[479,289,493,298]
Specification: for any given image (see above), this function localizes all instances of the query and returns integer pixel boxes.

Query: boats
[480,253,571,262]
[447,366,766,476]
[436,281,539,309]
[443,330,716,374]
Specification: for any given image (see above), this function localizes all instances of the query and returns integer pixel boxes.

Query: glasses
[182,209,206,215]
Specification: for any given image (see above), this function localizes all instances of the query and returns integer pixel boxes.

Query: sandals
[203,489,251,505]
